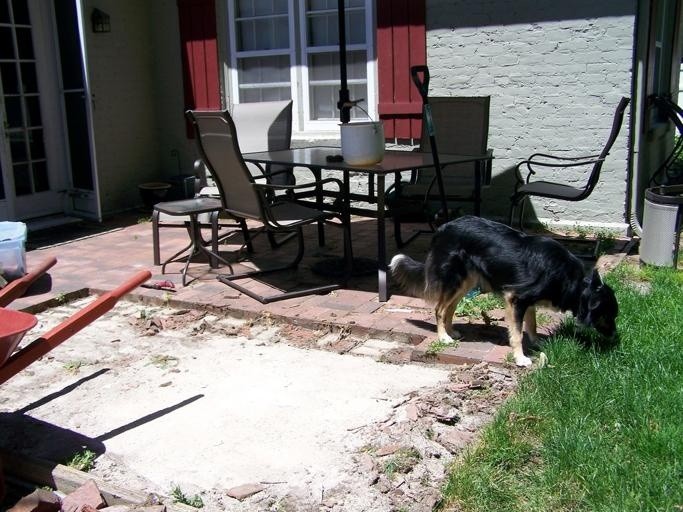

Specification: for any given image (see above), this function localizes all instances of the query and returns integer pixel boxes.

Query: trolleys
[0,254,152,385]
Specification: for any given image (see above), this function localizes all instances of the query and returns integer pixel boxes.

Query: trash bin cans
[639,184,683,270]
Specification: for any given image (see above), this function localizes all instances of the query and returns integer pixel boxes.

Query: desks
[240,146,494,303]
[153,197,234,287]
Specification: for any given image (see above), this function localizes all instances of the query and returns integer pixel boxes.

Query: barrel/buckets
[338,121,385,166]
[0,221,27,276]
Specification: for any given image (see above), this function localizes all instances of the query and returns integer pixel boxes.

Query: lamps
[89,8,110,34]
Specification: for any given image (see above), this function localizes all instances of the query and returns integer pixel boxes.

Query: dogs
[388,213,619,369]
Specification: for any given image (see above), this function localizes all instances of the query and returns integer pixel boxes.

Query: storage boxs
[0,220,27,287]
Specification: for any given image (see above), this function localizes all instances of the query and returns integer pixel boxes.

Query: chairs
[182,108,344,305]
[380,95,491,250]
[507,94,630,261]
[151,98,294,269]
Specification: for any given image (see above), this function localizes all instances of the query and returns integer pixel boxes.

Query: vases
[137,182,171,212]
